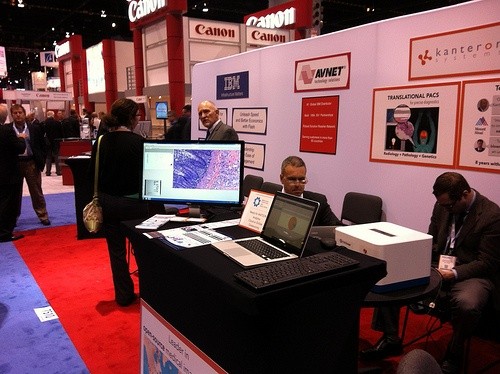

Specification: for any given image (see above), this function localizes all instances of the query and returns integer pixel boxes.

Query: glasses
[286,177,309,184]
[437,200,457,211]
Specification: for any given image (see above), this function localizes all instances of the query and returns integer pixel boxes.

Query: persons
[360,173,500,374]
[397,348,443,374]
[27,110,62,176]
[61,109,106,138]
[280,156,346,225]
[0,105,28,242]
[91,98,145,305]
[166,105,193,143]
[7,104,51,226]
[198,100,239,140]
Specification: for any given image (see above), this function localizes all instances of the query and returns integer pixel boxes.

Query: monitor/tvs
[139,139,245,208]
[155,101,168,120]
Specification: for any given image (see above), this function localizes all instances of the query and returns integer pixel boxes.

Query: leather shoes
[360,335,403,361]
[443,335,464,374]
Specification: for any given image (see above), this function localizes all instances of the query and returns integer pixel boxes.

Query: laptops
[210,190,320,269]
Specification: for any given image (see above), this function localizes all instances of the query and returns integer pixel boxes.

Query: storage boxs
[334,220,434,286]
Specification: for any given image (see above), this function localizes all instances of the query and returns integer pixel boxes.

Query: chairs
[243,174,263,197]
[401,307,500,374]
[341,192,382,224]
[260,181,282,194]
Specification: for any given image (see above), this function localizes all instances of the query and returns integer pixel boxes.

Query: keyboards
[232,251,360,292]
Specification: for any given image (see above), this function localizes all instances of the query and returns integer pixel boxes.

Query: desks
[360,267,441,306]
[121,206,387,374]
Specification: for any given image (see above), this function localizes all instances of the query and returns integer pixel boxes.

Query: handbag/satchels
[82,197,104,236]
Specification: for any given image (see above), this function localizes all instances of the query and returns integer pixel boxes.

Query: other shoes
[7,236,16,242]
[44,221,51,226]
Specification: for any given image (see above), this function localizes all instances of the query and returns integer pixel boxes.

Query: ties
[445,208,467,255]
[205,131,210,140]
